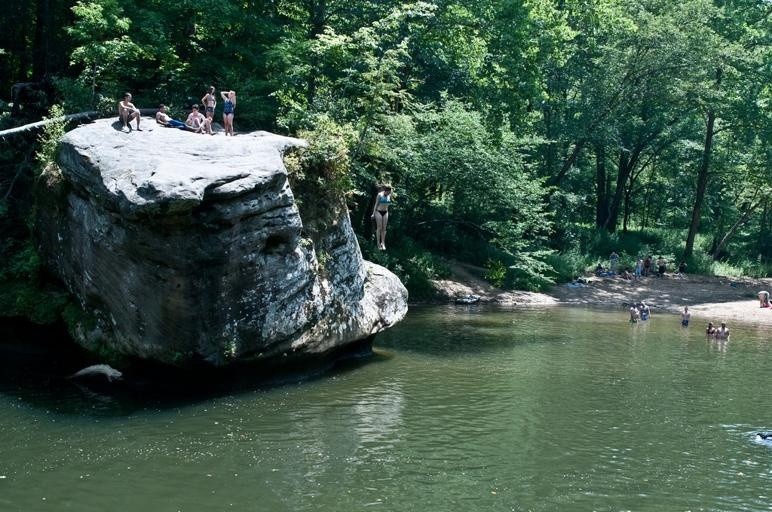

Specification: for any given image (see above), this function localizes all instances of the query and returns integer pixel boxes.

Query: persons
[371,185,393,250]
[758,290,772,309]
[681,307,690,326]
[706,322,729,340]
[594,251,667,280]
[118,92,143,133]
[156,86,217,135]
[678,260,688,280]
[629,301,650,323]
[220,90,237,137]
